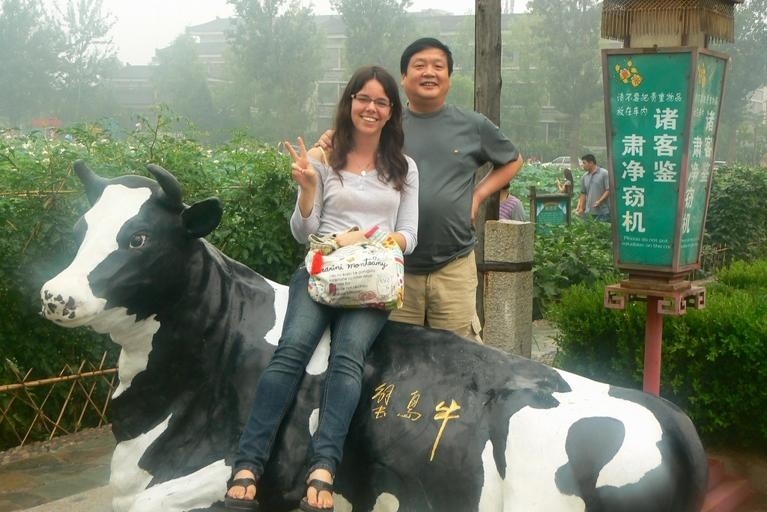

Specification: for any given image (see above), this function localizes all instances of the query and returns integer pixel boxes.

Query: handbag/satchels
[304,224,405,311]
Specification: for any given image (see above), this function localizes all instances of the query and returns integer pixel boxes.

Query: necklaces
[350,151,374,177]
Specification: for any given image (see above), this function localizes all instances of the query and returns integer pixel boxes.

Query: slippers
[224,475,259,510]
[299,479,335,512]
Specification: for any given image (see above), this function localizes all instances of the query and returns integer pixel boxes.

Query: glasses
[350,94,393,108]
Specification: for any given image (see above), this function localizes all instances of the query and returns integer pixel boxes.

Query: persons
[222,65,419,511]
[315,38,523,346]
[555,169,573,197]
[500,183,525,220]
[577,154,610,221]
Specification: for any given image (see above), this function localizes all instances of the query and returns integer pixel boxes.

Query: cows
[39,158,708,512]
